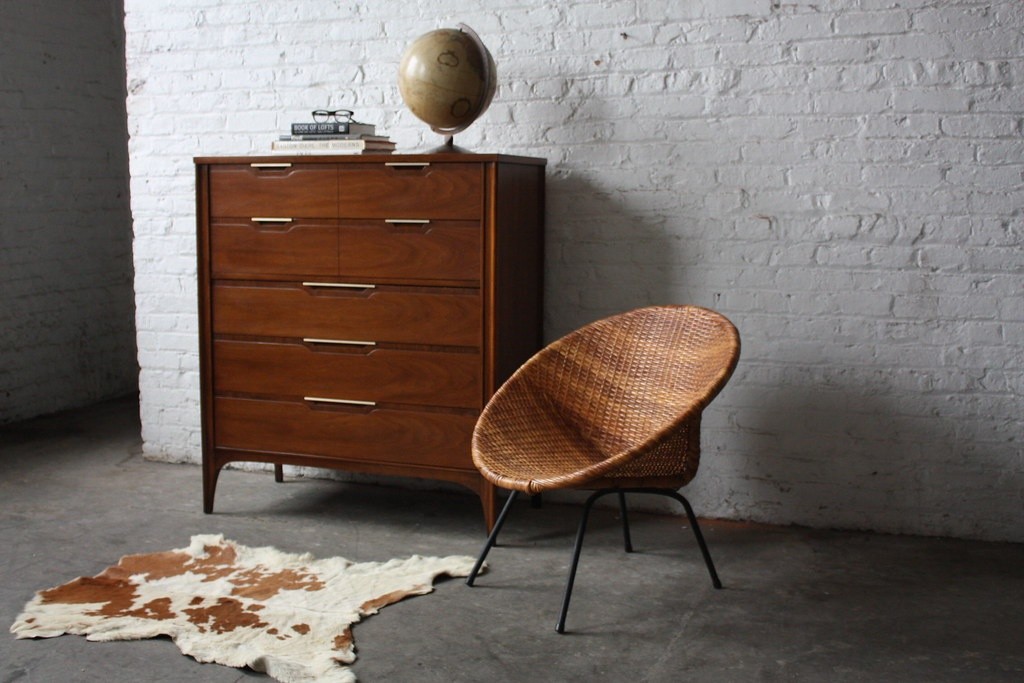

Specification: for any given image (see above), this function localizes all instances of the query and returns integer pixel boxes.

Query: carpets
[9,531,487,683]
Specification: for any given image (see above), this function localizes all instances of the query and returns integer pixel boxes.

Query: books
[247,123,397,156]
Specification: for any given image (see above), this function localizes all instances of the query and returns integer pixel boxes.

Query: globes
[397,23,498,154]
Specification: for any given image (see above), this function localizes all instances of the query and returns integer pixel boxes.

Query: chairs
[464,305,741,633]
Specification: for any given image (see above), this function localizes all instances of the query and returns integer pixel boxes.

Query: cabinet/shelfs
[194,155,548,546]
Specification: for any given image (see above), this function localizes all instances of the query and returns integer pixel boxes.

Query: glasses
[312,109,357,125]
